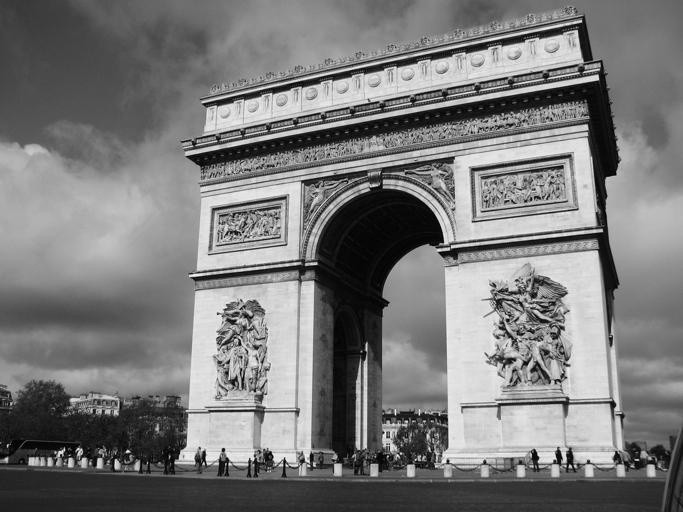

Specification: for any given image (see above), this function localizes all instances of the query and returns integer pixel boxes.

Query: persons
[213,307,262,398]
[555,447,563,472]
[318,452,324,469]
[219,448,228,474]
[310,451,314,471]
[298,451,305,464]
[53,445,108,468]
[531,449,539,472]
[566,447,577,472]
[351,449,442,475]
[613,448,671,472]
[34,448,40,458]
[170,454,175,474]
[252,448,274,477]
[487,267,565,386]
[194,446,208,474]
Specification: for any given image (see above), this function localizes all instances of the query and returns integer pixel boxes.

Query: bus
[0,439,81,467]
